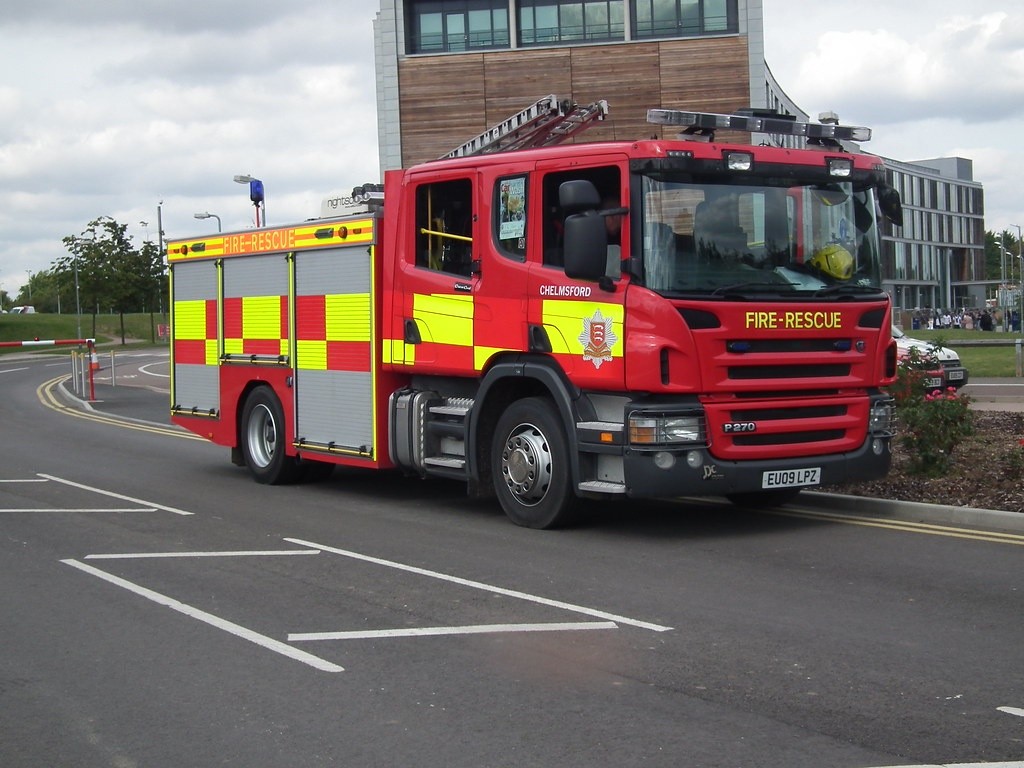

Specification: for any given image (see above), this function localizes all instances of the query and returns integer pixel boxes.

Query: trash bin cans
[913,318,920,329]
[1005,311,1019,331]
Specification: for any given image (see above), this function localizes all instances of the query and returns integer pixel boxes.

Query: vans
[10,306,35,313]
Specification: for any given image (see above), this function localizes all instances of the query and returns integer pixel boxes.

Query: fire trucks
[166,93,904,531]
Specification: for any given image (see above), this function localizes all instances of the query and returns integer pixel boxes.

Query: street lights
[74,242,92,349]
[1010,223,1024,333]
[193,212,221,232]
[25,269,32,300]
[232,173,266,227]
[993,235,1013,332]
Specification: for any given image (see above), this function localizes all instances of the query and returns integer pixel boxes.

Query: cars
[891,323,969,395]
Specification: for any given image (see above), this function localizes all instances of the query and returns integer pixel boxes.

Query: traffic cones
[91,346,105,371]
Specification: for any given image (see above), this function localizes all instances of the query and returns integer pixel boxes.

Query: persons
[601,192,622,246]
[932,307,998,333]
